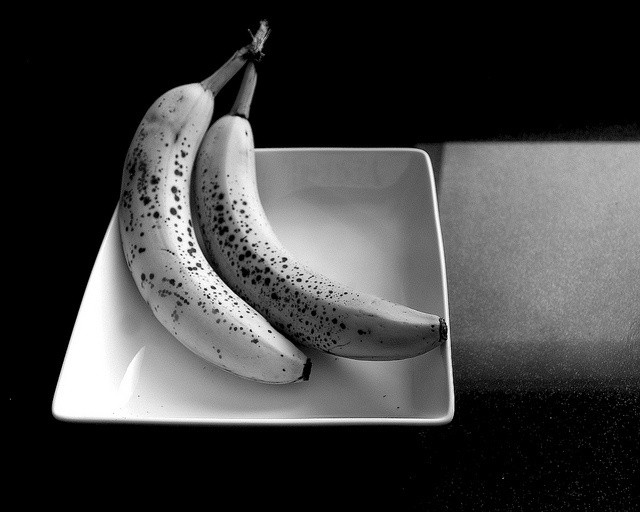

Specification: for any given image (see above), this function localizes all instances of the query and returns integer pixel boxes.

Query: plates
[52,148,455,425]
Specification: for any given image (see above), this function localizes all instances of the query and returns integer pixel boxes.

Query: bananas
[192,51,448,357]
[119,46,312,385]
